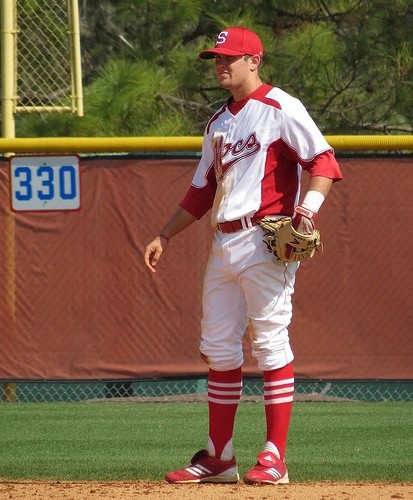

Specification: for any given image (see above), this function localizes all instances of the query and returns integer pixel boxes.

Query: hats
[199,27,264,60]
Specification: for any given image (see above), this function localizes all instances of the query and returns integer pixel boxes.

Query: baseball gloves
[259,216,324,264]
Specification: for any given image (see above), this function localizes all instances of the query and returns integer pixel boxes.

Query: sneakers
[242,451,290,485]
[165,449,240,484]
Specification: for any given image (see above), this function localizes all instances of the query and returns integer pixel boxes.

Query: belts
[216,218,263,233]
